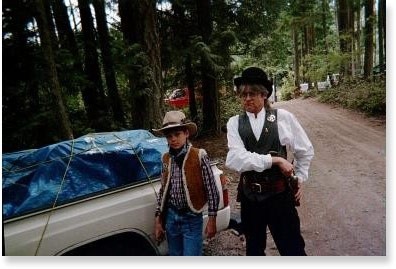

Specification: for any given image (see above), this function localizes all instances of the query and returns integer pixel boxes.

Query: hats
[150,111,197,137]
[234,67,273,98]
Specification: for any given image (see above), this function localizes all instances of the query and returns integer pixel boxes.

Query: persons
[225,66,314,256]
[151,111,220,256]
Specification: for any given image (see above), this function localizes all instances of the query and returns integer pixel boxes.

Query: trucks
[299,73,346,94]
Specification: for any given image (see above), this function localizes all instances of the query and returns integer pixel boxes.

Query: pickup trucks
[162,87,203,112]
[2,129,231,256]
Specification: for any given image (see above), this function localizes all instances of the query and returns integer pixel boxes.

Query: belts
[241,178,289,193]
